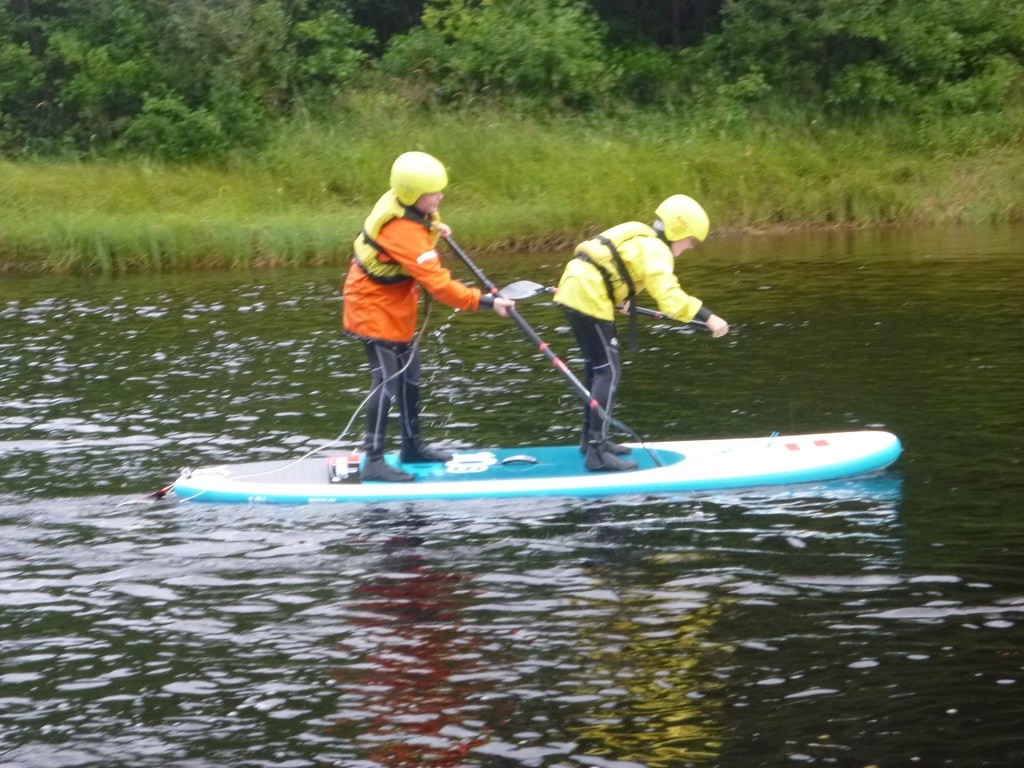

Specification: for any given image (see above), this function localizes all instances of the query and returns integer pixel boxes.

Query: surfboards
[172,424,903,506]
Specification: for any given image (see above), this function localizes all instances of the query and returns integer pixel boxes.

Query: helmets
[654,194,710,242]
[389,151,449,207]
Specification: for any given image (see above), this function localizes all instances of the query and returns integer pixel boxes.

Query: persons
[342,151,515,482]
[552,193,730,472]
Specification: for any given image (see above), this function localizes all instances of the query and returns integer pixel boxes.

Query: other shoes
[400,444,453,463]
[580,434,631,456]
[585,443,639,472]
[361,458,416,481]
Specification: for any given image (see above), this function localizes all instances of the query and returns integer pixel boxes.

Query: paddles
[443,232,660,469]
[496,278,712,328]
